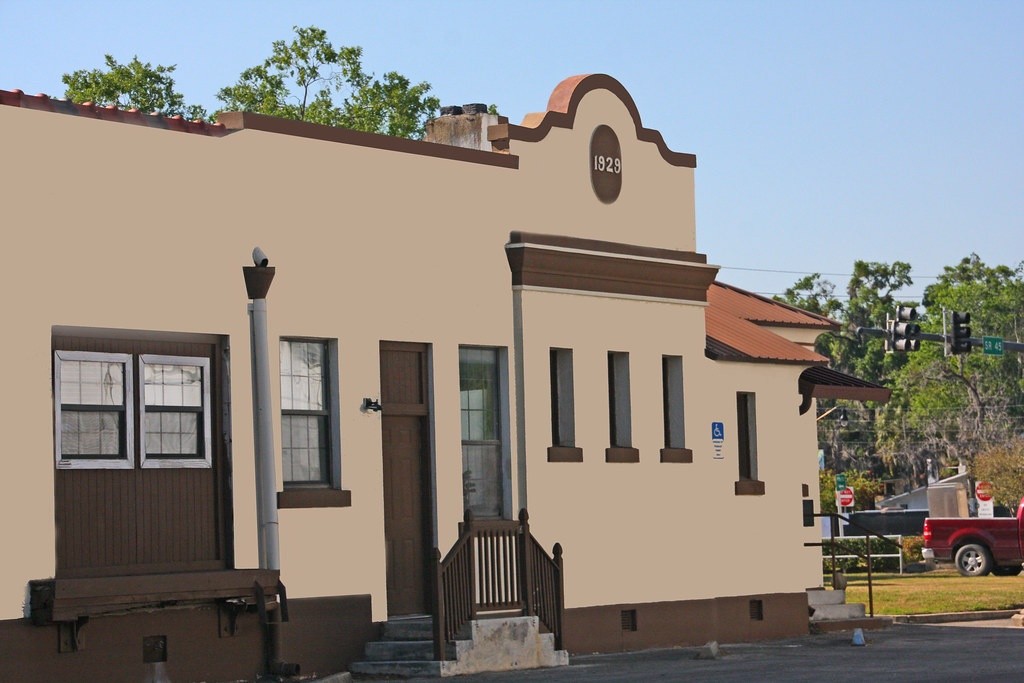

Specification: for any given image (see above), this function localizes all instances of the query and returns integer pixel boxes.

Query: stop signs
[835,487,855,507]
[975,481,995,503]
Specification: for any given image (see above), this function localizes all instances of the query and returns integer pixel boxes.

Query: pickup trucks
[921,496,1024,578]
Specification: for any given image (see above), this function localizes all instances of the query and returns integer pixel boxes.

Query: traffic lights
[907,323,922,355]
[943,308,973,358]
[891,305,916,357]
